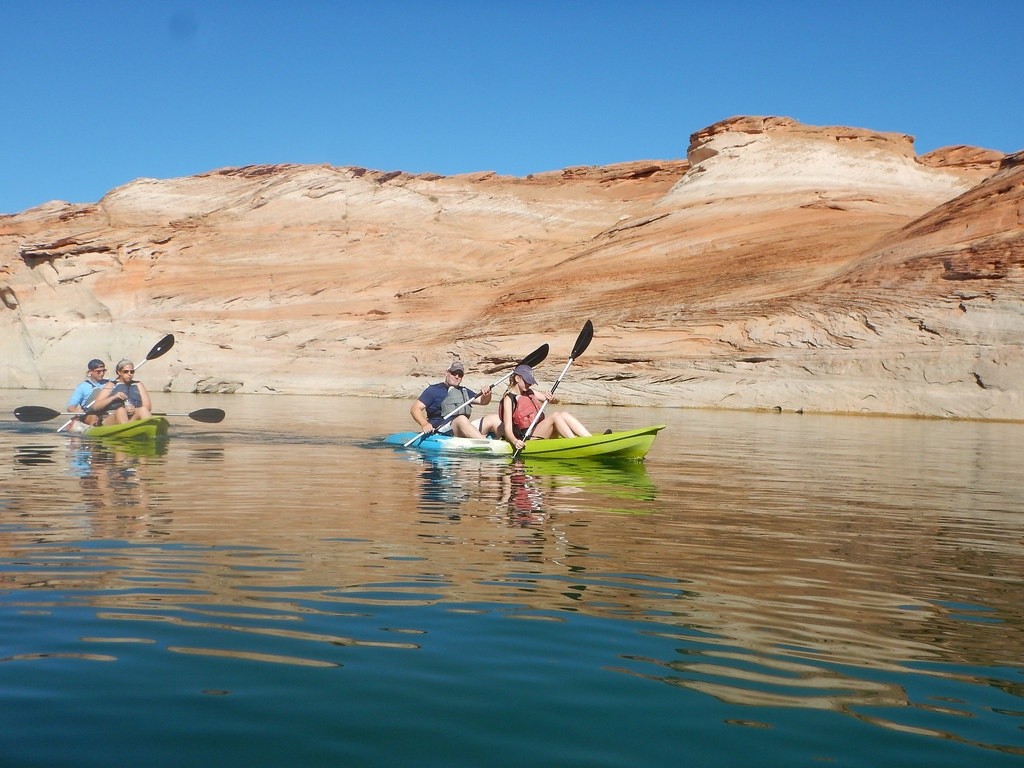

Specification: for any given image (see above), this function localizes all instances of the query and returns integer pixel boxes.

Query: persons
[411,362,502,440]
[66,359,116,426]
[499,365,612,450]
[92,359,152,426]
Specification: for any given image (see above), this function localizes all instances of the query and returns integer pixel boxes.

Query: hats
[88,359,105,371]
[448,361,464,374]
[514,365,538,385]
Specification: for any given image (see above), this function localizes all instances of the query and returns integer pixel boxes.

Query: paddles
[513,319,594,459]
[56,333,176,433]
[12,405,226,424]
[403,343,550,448]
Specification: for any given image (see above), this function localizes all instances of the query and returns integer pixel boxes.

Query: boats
[381,423,667,461]
[66,417,169,440]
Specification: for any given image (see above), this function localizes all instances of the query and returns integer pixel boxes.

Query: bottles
[486,429,496,439]
[124,396,132,411]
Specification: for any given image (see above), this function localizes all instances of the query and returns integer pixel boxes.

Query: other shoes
[604,429,612,435]
[499,435,506,440]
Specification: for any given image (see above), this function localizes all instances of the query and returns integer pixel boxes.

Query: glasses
[95,369,108,373]
[449,370,463,378]
[121,369,135,374]
